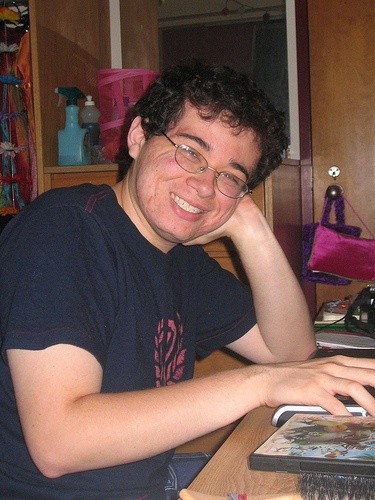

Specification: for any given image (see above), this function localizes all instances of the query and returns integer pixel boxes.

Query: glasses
[162,131,253,199]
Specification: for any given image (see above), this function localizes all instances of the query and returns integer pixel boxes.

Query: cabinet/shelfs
[44,157,303,457]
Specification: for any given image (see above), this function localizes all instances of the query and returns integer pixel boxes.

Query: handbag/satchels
[95,67,160,163]
[301,187,362,286]
[306,190,375,283]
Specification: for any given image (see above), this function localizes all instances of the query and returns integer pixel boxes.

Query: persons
[0,59,375,500]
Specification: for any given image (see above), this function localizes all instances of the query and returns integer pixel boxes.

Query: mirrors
[109,0,301,160]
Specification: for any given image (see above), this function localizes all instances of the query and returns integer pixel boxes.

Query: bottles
[81,95,102,163]
